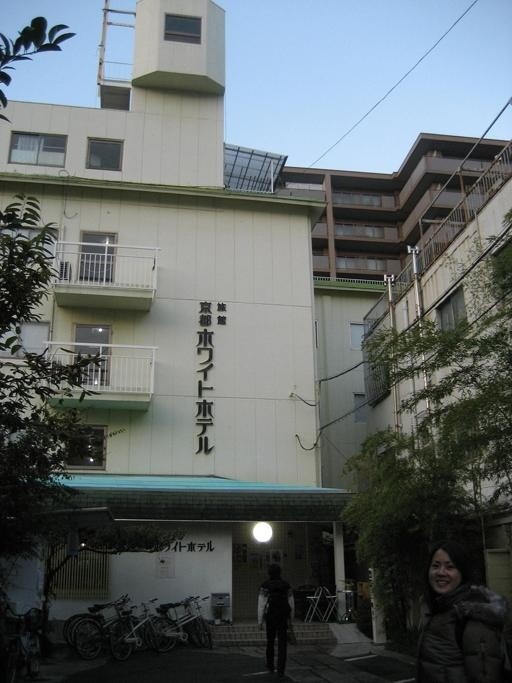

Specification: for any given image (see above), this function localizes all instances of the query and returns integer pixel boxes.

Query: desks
[337,590,362,622]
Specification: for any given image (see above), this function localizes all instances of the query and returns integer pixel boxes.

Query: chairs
[304,584,338,622]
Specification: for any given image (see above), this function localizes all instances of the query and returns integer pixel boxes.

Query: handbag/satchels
[287,630,297,645]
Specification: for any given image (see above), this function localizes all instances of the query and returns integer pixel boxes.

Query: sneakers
[265,664,284,671]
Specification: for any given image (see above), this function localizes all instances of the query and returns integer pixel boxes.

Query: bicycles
[63,593,214,662]
[5,605,44,683]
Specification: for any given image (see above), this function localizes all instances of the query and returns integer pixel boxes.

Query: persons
[256,562,296,682]
[414,539,512,682]
